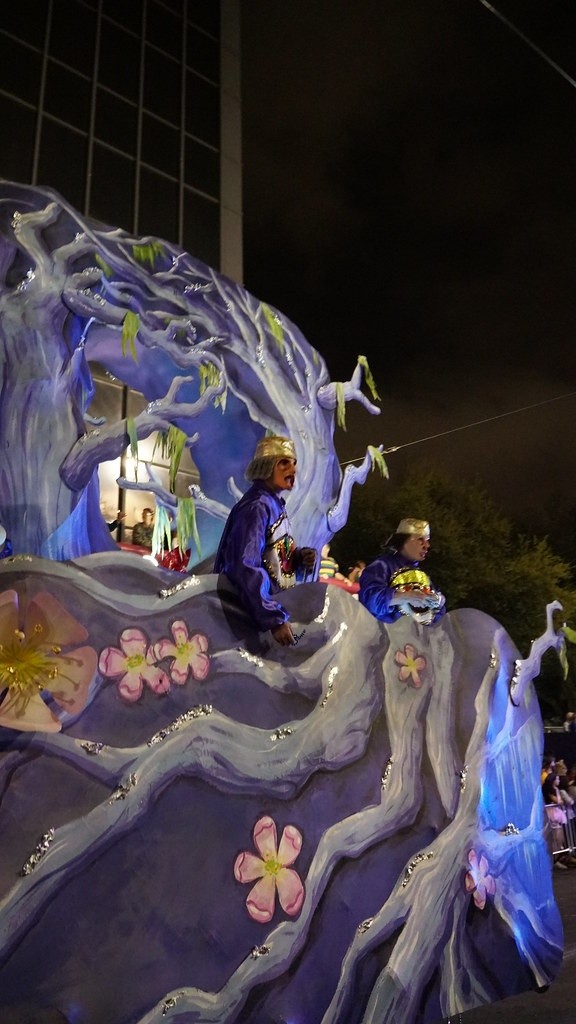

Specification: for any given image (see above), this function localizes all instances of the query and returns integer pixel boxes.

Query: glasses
[141,513,153,516]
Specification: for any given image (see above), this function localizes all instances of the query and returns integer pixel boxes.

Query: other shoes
[554,862,567,871]
[561,855,576,865]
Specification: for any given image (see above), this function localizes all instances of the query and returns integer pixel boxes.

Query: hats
[255,437,296,459]
[396,518,430,536]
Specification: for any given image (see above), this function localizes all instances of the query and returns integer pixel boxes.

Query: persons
[541,759,576,870]
[359,518,446,626]
[563,712,576,732]
[213,436,317,647]
[108,508,155,548]
